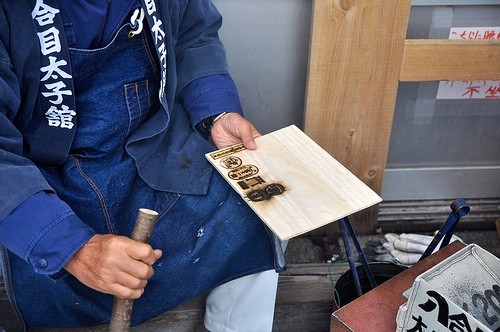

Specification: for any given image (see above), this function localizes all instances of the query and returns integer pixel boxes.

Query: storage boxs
[330,239,500,332]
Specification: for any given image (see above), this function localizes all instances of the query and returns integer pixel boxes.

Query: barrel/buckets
[333,262,413,309]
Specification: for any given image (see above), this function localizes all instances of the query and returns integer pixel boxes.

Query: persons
[0,0,287,332]
